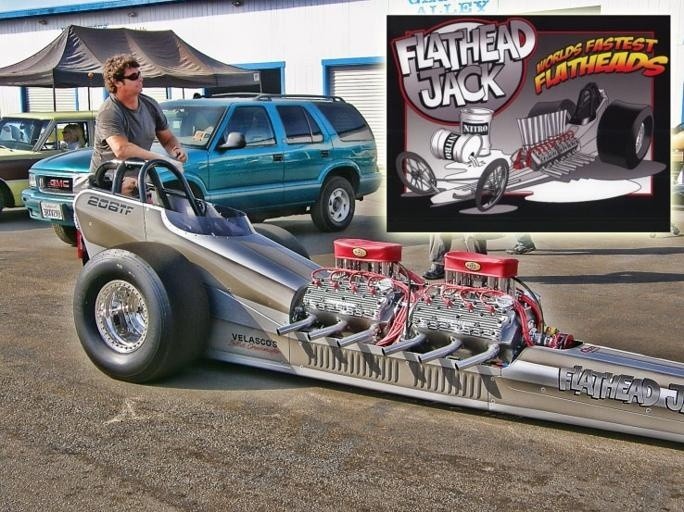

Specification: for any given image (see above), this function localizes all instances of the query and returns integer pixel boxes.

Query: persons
[81,53,189,269]
[61,124,86,152]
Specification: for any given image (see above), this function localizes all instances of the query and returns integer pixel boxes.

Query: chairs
[244,112,273,143]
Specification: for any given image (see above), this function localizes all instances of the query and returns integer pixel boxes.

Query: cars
[0,112,101,219]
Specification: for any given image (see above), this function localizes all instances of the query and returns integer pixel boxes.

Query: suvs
[24,90,386,249]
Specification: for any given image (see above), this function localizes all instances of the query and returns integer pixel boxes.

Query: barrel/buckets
[429,128,482,164]
[460,107,495,155]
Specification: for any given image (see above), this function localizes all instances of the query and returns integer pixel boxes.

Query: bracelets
[171,145,181,151]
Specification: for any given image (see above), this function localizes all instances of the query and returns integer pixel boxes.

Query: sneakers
[506,240,536,254]
[422,264,445,278]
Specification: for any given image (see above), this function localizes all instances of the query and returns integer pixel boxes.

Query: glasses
[116,71,142,81]
